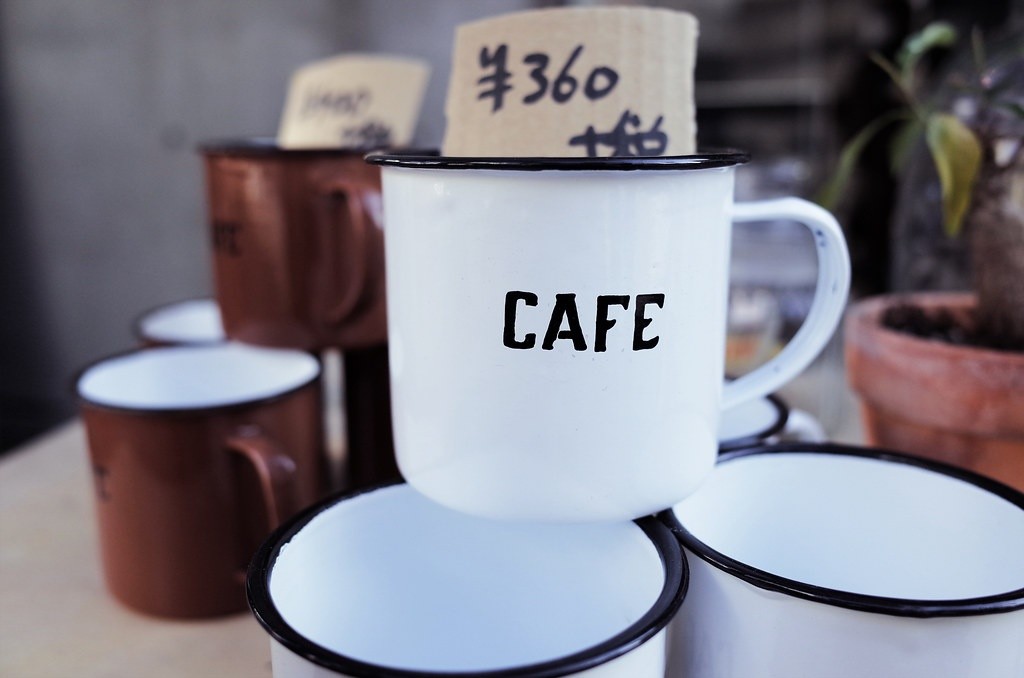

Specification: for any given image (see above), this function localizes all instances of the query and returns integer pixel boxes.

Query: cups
[74,136,1024,678]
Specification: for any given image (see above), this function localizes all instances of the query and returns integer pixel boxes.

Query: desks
[0,351,348,678]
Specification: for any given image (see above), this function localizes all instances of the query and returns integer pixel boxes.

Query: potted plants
[821,25,1023,491]
